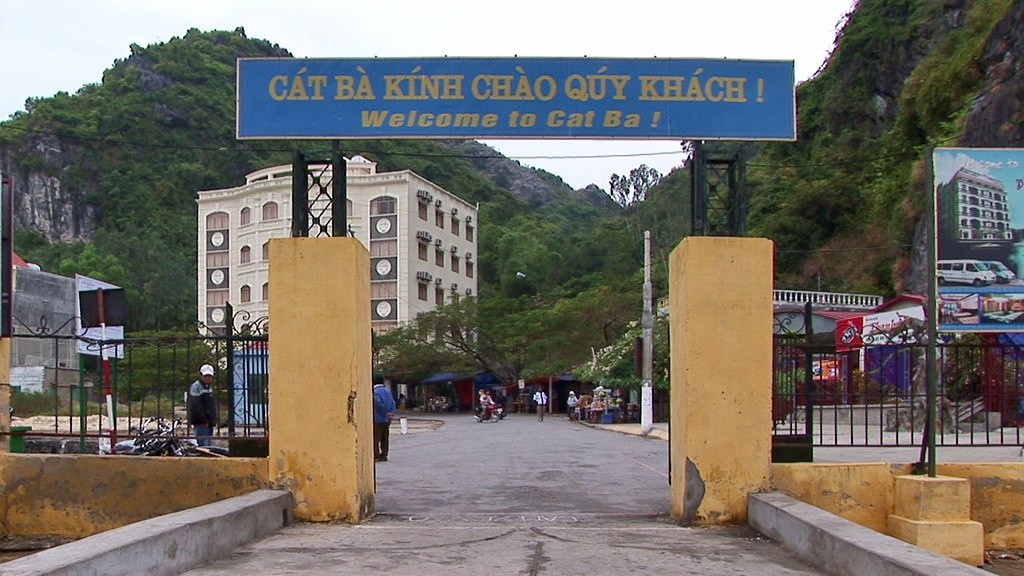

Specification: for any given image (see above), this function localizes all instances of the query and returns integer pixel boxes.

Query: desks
[513,401,528,412]
[574,408,640,423]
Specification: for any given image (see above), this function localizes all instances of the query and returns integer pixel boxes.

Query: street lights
[515,271,553,415]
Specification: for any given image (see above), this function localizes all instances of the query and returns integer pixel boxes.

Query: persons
[478,388,507,418]
[372,374,397,461]
[567,390,579,422]
[578,392,586,421]
[532,385,547,422]
[189,364,218,447]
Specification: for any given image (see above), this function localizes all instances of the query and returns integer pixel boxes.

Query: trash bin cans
[602,413,614,424]
[9,426,31,453]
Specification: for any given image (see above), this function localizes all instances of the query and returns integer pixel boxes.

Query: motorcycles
[473,397,507,423]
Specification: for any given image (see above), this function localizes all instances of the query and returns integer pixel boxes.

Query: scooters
[97,415,230,459]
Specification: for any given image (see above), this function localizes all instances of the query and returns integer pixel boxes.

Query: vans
[937,259,997,288]
[974,261,1017,285]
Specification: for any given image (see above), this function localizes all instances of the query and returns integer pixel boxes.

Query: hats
[200,365,214,376]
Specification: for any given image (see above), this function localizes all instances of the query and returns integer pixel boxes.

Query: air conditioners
[466,216,471,221]
[437,278,442,284]
[467,288,472,294]
[418,230,432,241]
[436,239,442,246]
[417,271,433,281]
[452,208,458,214]
[452,283,458,289]
[467,252,471,258]
[417,190,433,201]
[436,199,442,206]
[452,246,457,252]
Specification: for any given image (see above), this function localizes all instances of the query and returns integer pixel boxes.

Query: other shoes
[380,453,388,461]
[374,455,379,462]
